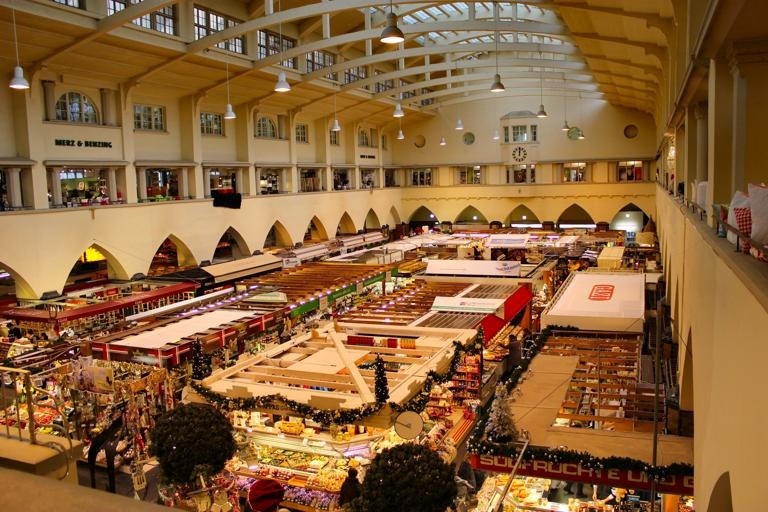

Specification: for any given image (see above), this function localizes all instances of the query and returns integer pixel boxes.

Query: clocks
[512,147,527,162]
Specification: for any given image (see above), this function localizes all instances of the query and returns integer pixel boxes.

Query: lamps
[378,4,405,45]
[328,77,343,134]
[272,4,294,95]
[493,107,501,142]
[535,51,548,120]
[224,32,238,121]
[396,120,406,140]
[578,118,584,140]
[454,97,464,132]
[391,53,406,119]
[10,1,29,90]
[490,33,506,94]
[439,124,447,146]
[562,86,570,132]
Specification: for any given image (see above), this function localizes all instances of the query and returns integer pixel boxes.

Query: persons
[631,166,634,180]
[655,168,659,182]
[6,319,74,344]
[47,189,53,205]
[668,174,674,195]
[266,174,277,196]
